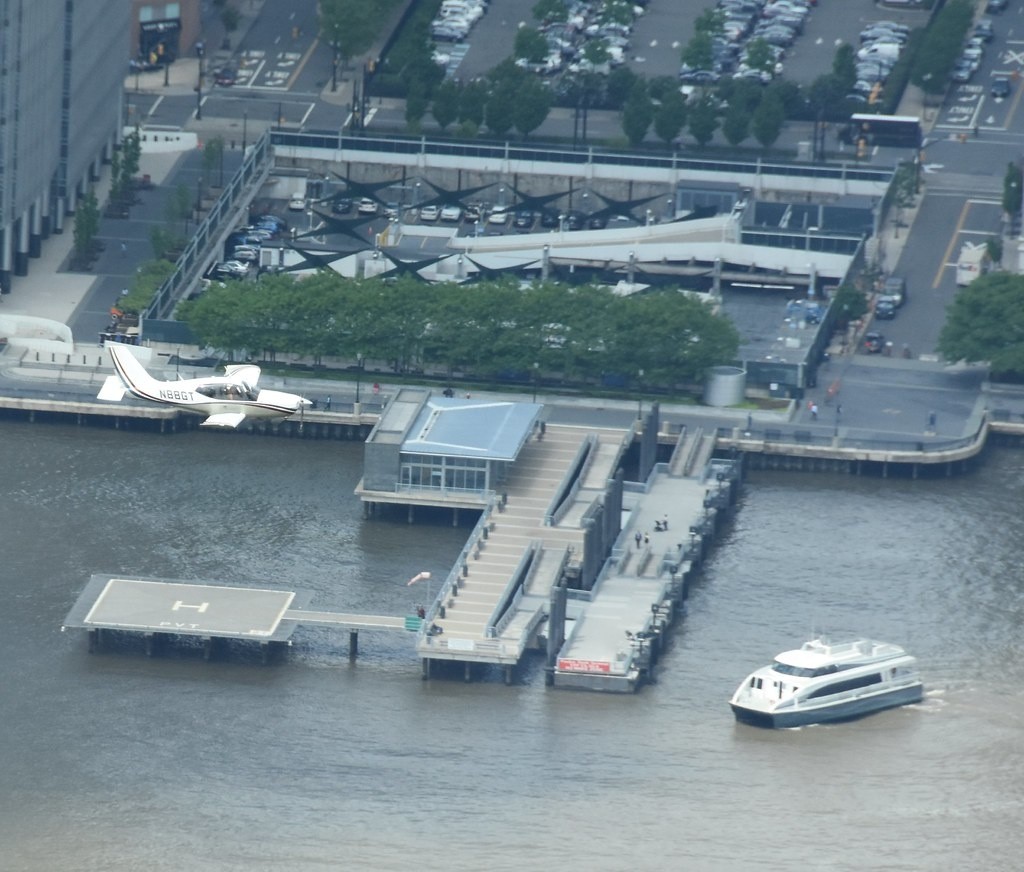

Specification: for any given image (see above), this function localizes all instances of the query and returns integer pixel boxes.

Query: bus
[850,114,920,147]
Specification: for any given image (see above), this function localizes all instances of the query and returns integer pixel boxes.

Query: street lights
[196,42,202,119]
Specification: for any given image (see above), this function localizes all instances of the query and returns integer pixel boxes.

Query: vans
[957,247,985,286]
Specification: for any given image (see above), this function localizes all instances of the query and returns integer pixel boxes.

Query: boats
[729,634,923,727]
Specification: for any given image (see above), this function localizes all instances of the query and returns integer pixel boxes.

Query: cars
[861,331,883,352]
[948,22,991,82]
[674,0,812,97]
[428,0,489,42]
[874,277,906,320]
[288,192,306,212]
[989,0,1007,13]
[846,19,909,104]
[330,193,607,228]
[513,0,643,100]
[216,214,287,280]
[991,77,1010,97]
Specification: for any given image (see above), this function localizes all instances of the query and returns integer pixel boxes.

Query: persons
[467,392,470,399]
[323,394,331,411]
[926,410,936,432]
[120,288,128,297]
[972,120,979,137]
[635,513,670,548]
[807,400,819,418]
[120,242,127,257]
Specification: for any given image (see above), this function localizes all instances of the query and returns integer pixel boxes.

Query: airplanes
[110,345,313,431]
